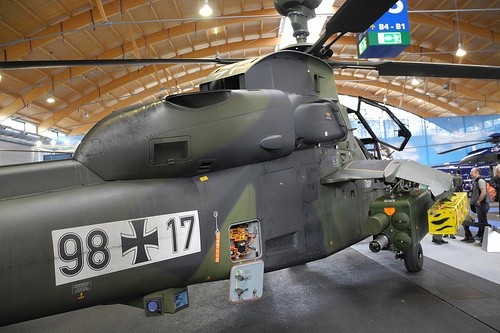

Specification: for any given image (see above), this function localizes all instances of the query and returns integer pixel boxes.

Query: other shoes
[443,235,447,237]
[466,238,475,243]
[460,237,468,241]
[473,234,482,240]
[450,234,455,239]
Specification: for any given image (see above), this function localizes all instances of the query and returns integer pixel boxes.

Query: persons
[494,164,500,217]
[461,220,475,243]
[453,170,463,192]
[443,234,456,239]
[470,168,490,246]
[431,192,448,245]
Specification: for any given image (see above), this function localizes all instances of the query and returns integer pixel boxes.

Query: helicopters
[0,0,500,331]
[430,133,500,203]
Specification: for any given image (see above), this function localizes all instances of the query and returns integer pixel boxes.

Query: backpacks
[476,177,497,202]
